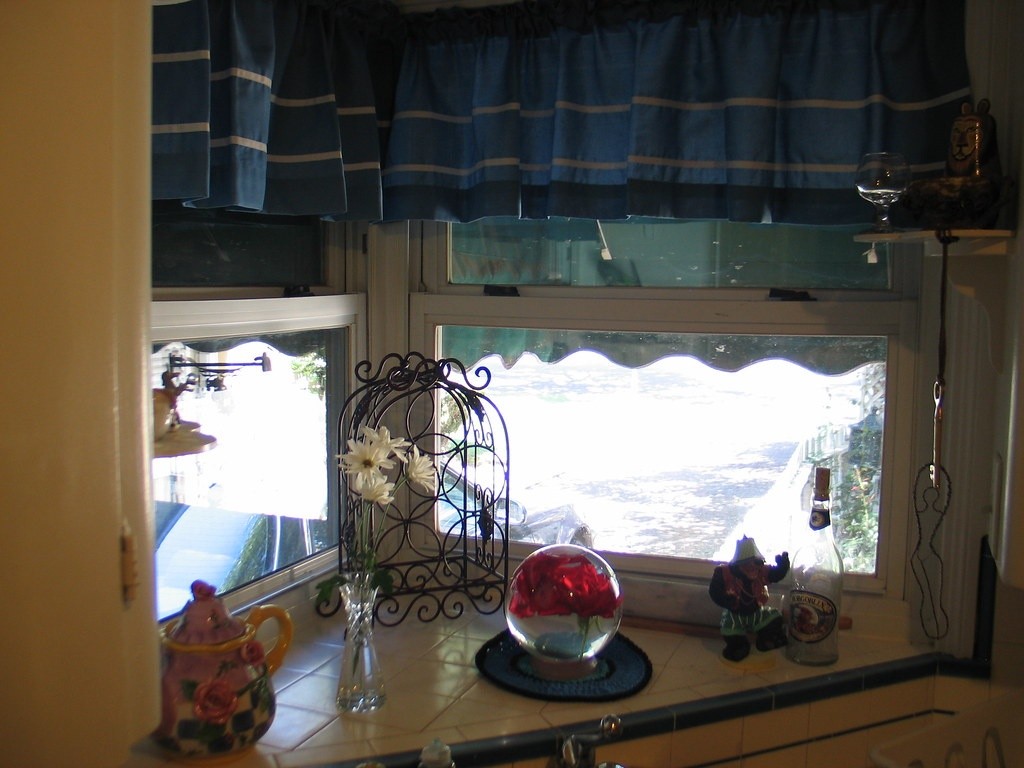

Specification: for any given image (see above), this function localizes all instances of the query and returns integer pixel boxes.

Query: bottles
[786,469,843,666]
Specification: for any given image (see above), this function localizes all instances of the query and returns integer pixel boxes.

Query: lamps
[152,352,273,460]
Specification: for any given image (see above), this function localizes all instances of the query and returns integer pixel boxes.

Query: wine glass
[855,152,907,234]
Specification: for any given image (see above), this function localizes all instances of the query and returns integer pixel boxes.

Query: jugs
[149,581,293,766]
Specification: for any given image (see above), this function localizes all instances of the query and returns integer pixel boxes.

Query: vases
[335,573,389,715]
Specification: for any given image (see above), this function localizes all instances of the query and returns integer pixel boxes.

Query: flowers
[192,678,238,726]
[241,639,264,667]
[508,551,623,662]
[313,426,437,609]
